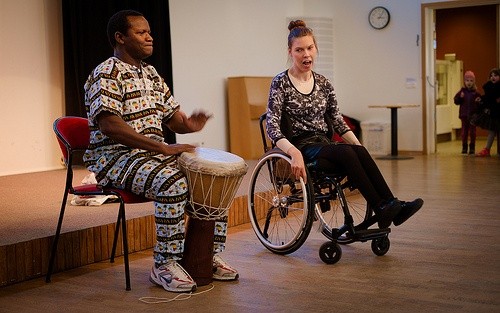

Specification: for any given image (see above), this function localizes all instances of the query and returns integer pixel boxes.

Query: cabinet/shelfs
[228,76,277,160]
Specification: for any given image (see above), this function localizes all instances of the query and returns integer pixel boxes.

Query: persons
[475,68,500,156]
[264,19,424,229]
[83,9,239,293]
[454,70,482,154]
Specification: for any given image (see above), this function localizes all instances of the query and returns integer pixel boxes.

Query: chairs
[45,116,155,293]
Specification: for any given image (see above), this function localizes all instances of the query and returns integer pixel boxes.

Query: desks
[367,103,421,161]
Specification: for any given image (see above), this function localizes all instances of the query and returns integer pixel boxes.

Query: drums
[174,145,249,290]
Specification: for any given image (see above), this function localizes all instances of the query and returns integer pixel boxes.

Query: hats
[464,71,475,81]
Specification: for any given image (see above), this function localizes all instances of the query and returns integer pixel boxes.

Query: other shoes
[476,148,491,157]
[393,198,424,227]
[375,199,401,230]
[469,143,475,156]
[462,143,468,156]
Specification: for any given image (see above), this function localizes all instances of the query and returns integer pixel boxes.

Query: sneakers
[149,262,198,293]
[213,256,240,282]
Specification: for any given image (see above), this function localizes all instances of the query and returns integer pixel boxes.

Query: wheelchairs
[246,110,392,265]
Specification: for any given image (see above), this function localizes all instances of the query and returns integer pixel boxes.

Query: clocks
[368,6,391,30]
